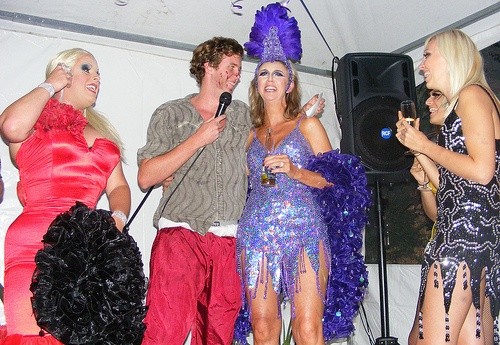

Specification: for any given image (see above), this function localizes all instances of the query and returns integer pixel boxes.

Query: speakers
[334,53,421,186]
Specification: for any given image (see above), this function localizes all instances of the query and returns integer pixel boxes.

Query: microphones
[215,92,232,118]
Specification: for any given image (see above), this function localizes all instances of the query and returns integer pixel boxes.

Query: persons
[163,63,369,345]
[137,37,325,345]
[0,48,131,345]
[410,91,449,221]
[395,30,500,345]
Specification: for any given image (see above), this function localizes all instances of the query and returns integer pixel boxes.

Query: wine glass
[400,99,419,154]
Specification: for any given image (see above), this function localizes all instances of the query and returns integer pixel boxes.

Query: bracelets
[417,180,430,187]
[111,210,128,225]
[38,82,54,97]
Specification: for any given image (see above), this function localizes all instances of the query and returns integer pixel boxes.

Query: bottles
[261,128,276,187]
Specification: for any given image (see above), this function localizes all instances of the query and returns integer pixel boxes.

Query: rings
[65,66,70,72]
[280,162,284,167]
